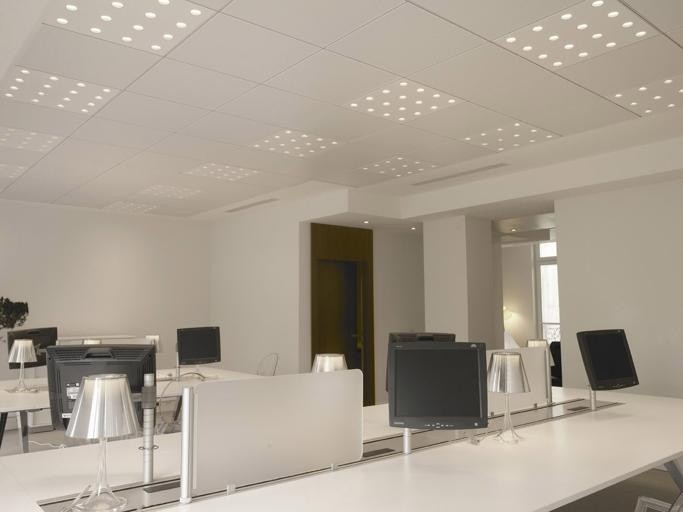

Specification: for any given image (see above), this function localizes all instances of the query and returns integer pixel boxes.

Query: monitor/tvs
[46,344,156,431]
[177,327,220,365]
[8,327,57,369]
[386,332,455,392]
[577,329,639,390]
[388,341,488,430]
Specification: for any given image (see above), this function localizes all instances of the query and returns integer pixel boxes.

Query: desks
[0,365,682,510]
